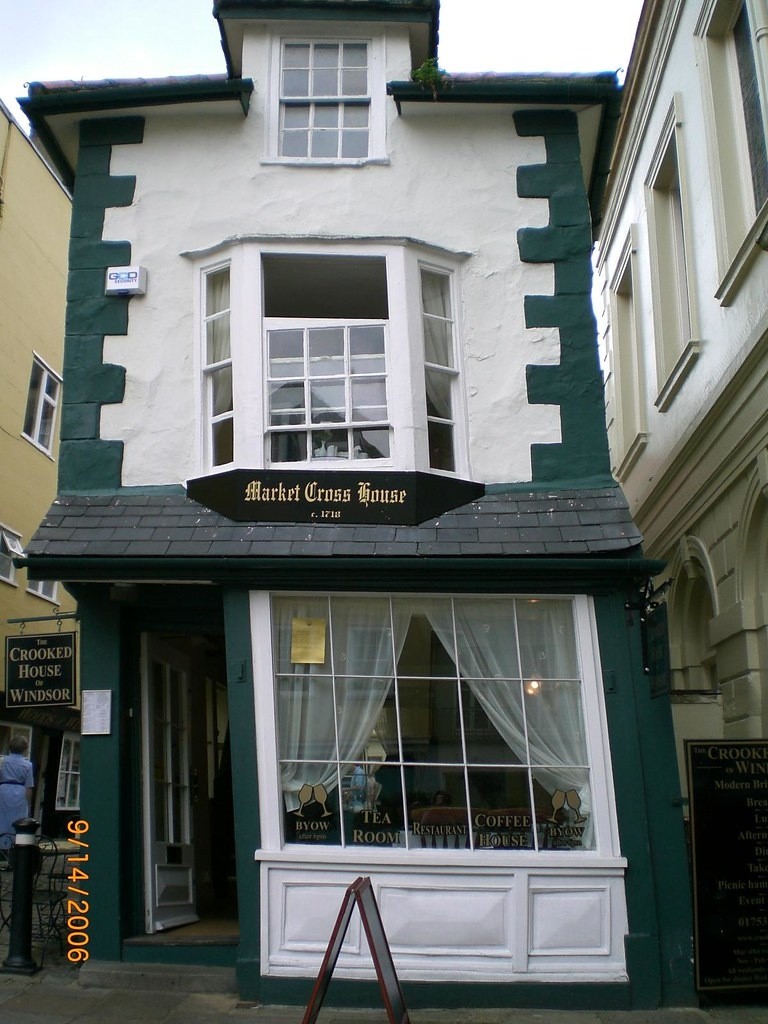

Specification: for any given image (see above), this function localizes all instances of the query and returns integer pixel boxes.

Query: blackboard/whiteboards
[296,875,417,1024]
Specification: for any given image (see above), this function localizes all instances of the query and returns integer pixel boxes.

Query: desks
[32,839,81,939]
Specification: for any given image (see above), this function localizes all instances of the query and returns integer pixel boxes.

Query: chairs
[0,832,81,969]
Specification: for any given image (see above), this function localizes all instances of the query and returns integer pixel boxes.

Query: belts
[1,781,25,785]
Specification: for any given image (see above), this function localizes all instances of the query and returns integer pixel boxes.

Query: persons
[0,736,34,850]
[342,763,366,812]
